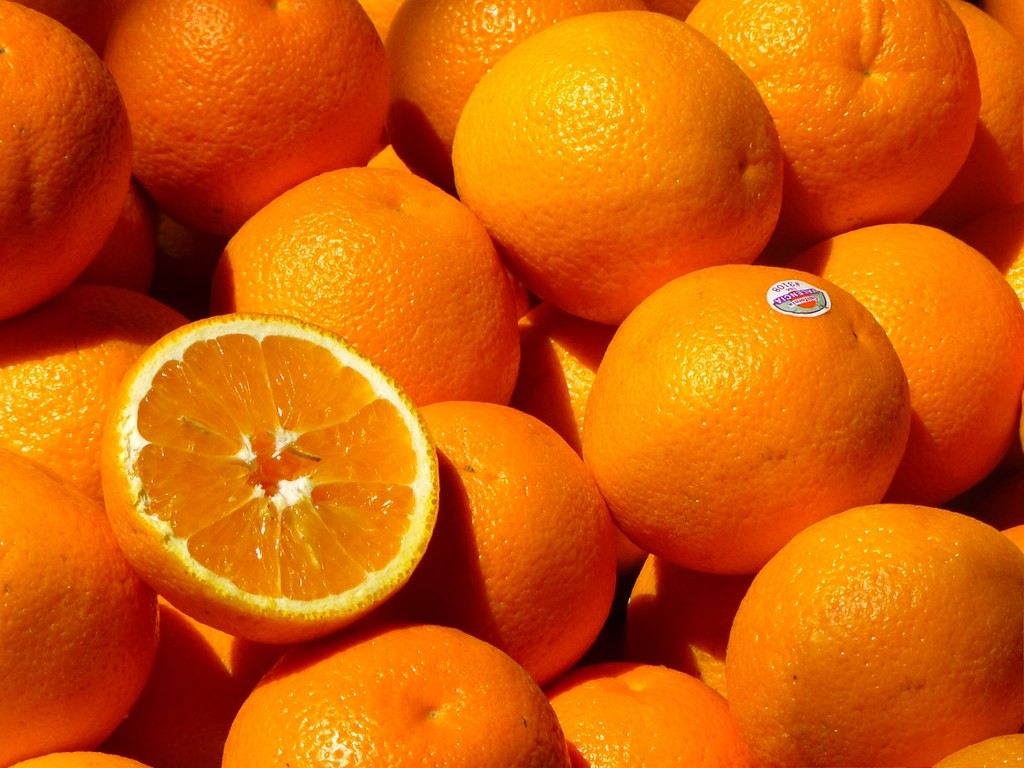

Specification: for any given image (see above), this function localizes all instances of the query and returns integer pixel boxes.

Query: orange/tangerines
[0,0,1024,767]
